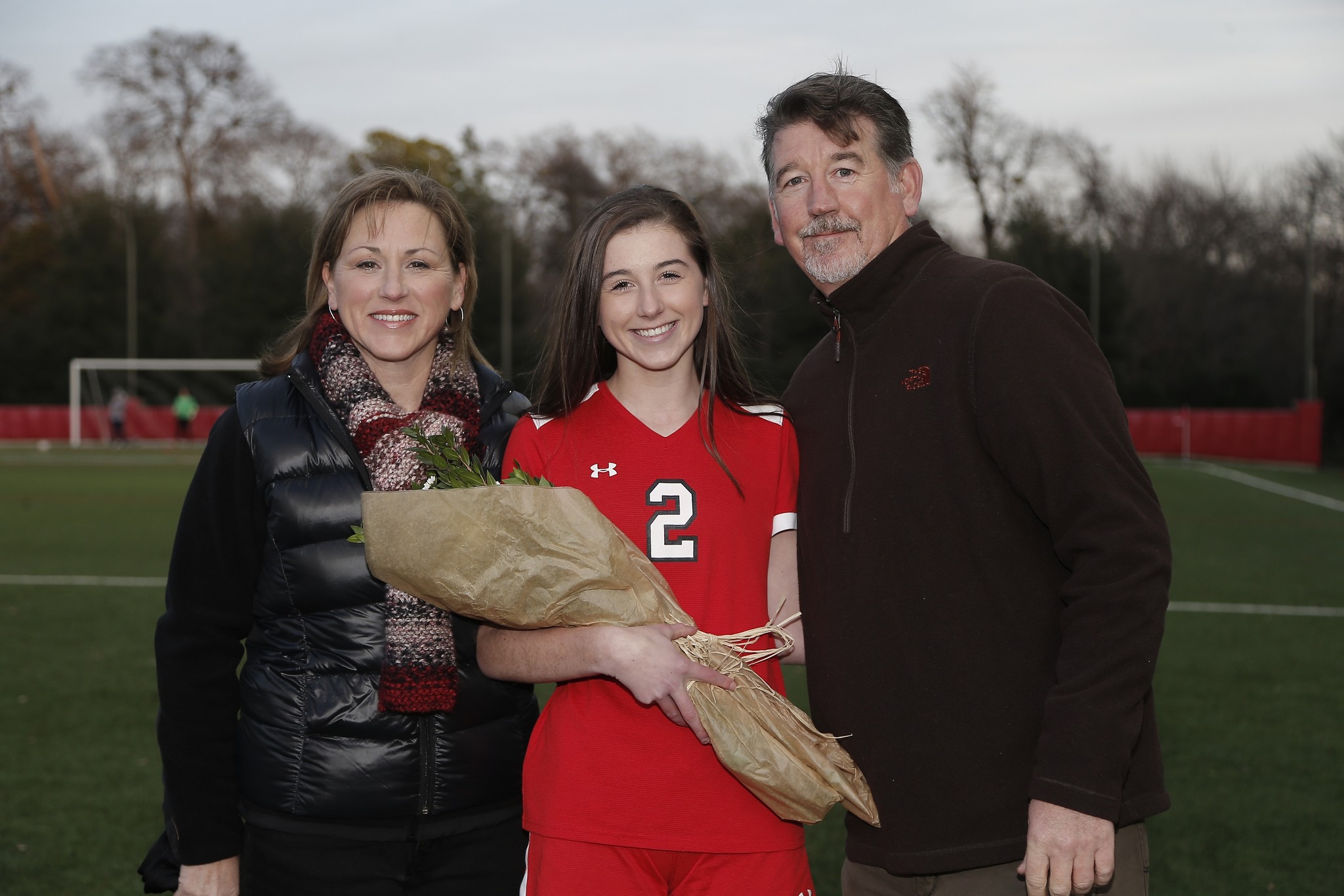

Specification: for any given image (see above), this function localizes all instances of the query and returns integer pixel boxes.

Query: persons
[106,386,129,443]
[474,186,809,893]
[750,73,1170,895]
[169,384,197,436]
[135,170,534,895]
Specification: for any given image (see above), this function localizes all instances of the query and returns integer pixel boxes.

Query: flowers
[344,416,559,545]
